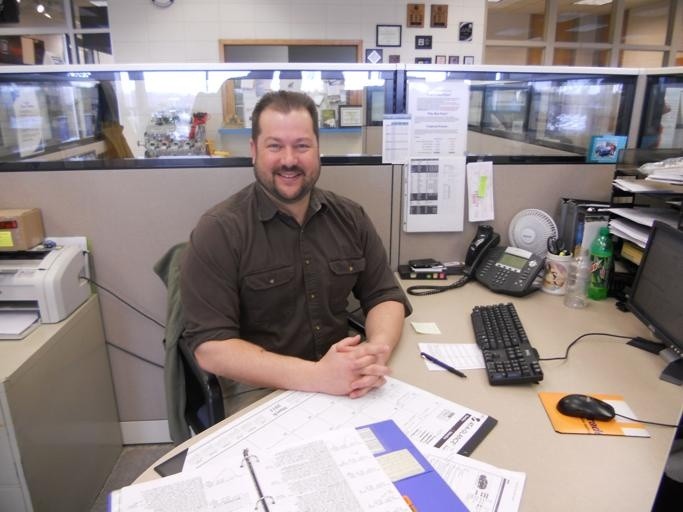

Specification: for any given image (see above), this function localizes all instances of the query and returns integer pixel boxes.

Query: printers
[0,235,94,339]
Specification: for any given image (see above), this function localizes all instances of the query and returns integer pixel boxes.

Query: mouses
[557,395,615,421]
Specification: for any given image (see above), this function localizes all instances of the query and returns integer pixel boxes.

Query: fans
[506,207,555,259]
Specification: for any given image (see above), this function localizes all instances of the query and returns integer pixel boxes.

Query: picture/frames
[363,22,477,68]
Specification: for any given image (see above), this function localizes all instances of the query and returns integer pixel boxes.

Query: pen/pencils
[421,352,467,378]
[559,249,571,256]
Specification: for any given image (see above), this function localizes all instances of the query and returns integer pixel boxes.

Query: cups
[543,252,570,294]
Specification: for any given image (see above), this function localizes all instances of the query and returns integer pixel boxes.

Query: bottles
[564,246,592,308]
[587,226,614,302]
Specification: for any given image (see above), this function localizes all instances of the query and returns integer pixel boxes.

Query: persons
[181,90,413,420]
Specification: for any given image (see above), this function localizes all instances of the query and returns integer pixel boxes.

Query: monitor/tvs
[621,218,683,386]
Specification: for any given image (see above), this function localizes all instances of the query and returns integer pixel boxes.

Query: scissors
[547,237,566,255]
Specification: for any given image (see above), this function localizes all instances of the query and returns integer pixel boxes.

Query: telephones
[462,233,546,298]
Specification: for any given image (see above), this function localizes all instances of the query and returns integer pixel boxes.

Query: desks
[112,262,683,511]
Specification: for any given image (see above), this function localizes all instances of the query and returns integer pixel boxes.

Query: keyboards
[471,302,543,386]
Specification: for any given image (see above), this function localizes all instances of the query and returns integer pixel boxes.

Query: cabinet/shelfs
[0,290,124,511]
[604,168,682,302]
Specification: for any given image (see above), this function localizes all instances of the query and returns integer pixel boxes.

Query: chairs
[152,242,230,433]
